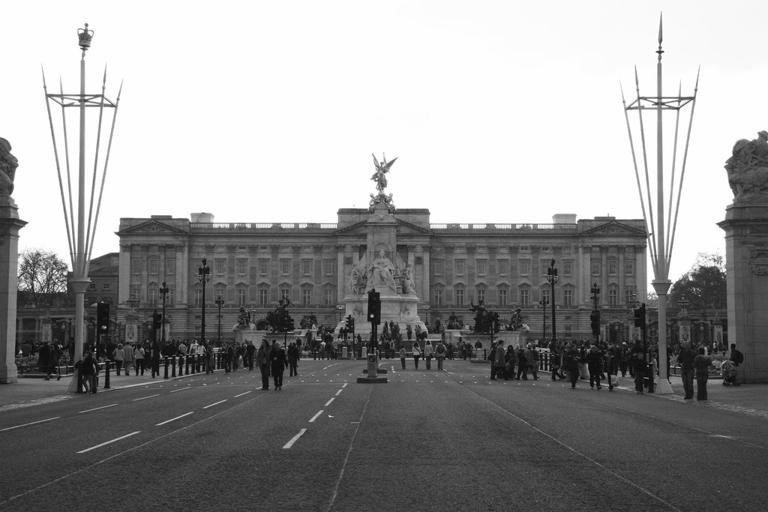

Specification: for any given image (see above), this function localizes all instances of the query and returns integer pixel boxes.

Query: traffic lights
[97,303,109,333]
[591,310,600,335]
[368,289,381,321]
[634,307,643,327]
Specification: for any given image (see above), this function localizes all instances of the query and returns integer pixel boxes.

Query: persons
[505,307,523,331]
[487,330,742,401]
[471,299,488,334]
[276,294,291,333]
[369,151,399,193]
[351,251,416,297]
[14,308,482,393]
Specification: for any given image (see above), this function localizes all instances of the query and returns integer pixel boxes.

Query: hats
[527,342,533,347]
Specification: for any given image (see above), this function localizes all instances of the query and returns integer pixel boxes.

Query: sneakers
[491,375,538,381]
[262,386,282,391]
[571,384,602,389]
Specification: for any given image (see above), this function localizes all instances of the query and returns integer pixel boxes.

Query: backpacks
[737,351,743,363]
[438,344,444,353]
[82,357,94,374]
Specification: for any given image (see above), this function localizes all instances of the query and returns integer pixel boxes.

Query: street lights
[215,295,224,342]
[591,282,600,309]
[160,280,169,341]
[540,258,557,340]
[199,258,209,343]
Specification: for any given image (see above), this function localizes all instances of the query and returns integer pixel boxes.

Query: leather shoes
[685,396,692,399]
